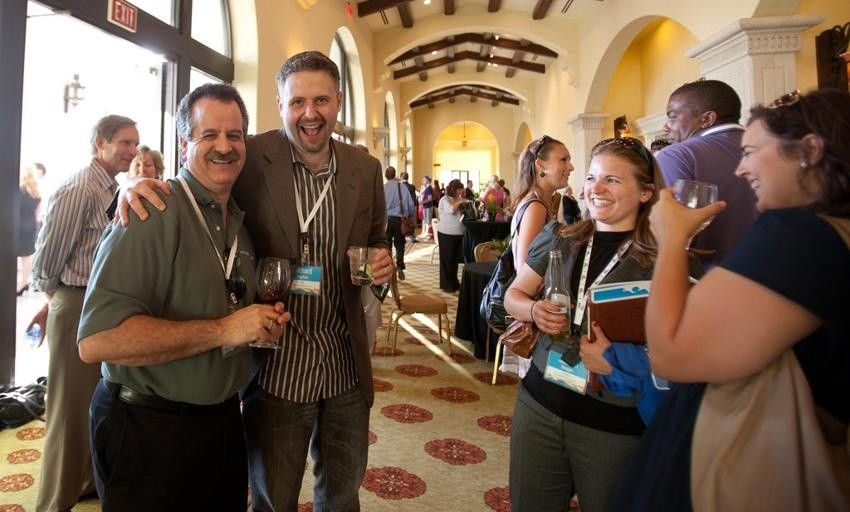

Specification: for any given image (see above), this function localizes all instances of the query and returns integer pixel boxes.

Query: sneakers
[396,266,404,280]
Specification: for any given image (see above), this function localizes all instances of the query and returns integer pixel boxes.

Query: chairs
[383,260,453,357]
[430,217,441,265]
[473,238,508,265]
[485,319,511,385]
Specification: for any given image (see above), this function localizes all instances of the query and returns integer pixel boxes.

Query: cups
[674,180,718,253]
[349,245,376,286]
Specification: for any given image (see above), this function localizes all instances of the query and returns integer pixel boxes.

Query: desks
[454,262,506,365]
[462,216,513,265]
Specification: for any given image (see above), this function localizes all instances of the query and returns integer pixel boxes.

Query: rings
[268,319,276,331]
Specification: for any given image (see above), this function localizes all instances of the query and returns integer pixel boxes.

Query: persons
[26,115,140,512]
[76,84,292,512]
[562,187,581,225]
[30,162,50,236]
[645,87,850,512]
[656,80,762,275]
[17,164,43,297]
[129,146,164,179]
[399,172,511,243]
[499,135,574,379]
[113,50,395,512]
[438,179,473,293]
[383,167,415,280]
[503,137,706,512]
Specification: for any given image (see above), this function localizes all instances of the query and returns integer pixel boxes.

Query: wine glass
[249,256,291,350]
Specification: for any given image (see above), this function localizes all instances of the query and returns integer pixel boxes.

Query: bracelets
[529,301,536,323]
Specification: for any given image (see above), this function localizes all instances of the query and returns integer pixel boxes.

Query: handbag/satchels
[401,216,415,236]
[691,346,850,511]
[1,378,48,428]
[479,249,541,359]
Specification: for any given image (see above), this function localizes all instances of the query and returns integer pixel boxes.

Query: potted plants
[491,238,508,261]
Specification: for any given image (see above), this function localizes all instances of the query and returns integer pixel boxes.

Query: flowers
[484,194,502,216]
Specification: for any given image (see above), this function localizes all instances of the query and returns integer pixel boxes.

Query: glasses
[534,134,552,161]
[222,240,247,301]
[592,137,651,175]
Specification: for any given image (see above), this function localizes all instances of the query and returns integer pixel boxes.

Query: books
[586,280,653,391]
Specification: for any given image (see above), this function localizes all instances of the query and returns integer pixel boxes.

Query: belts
[119,385,241,414]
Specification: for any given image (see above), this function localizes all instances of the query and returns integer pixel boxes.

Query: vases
[488,213,496,221]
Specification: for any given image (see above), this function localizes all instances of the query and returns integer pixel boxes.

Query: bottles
[546,250,572,341]
[23,324,41,347]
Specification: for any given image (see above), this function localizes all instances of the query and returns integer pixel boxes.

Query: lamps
[63,71,88,114]
